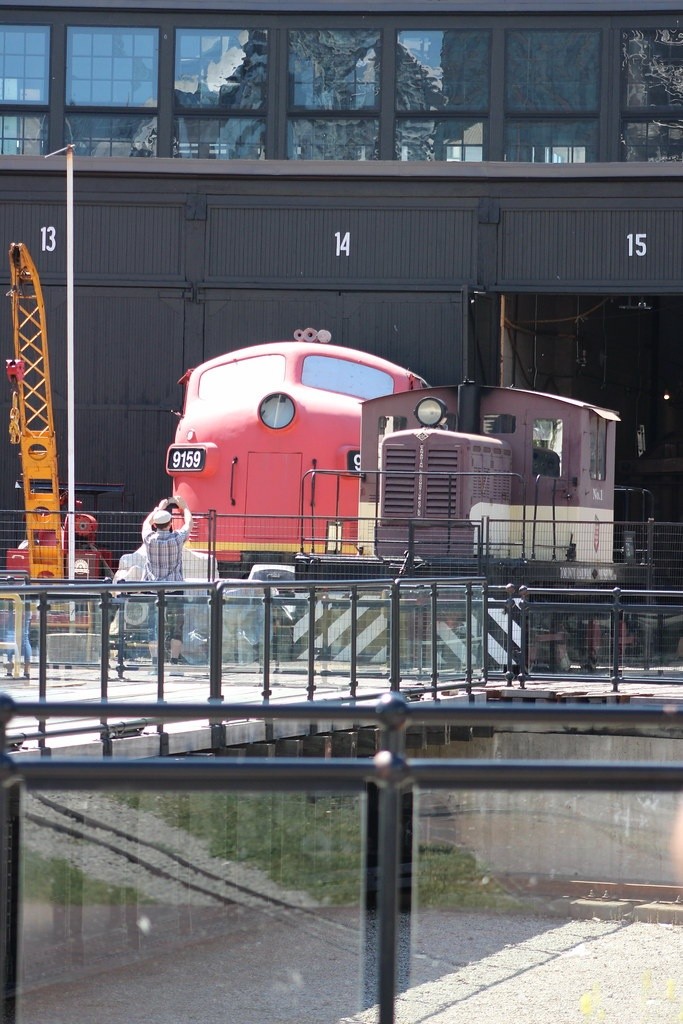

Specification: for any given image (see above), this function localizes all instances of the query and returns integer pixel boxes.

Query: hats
[153,510,172,524]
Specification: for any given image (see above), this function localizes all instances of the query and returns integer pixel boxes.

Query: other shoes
[150,671,158,675]
[169,669,184,675]
[5,673,29,679]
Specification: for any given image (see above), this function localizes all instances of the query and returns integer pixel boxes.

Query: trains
[291,379,682,672]
[106,327,451,664]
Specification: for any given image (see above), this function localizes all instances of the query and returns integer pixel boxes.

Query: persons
[141,496,198,676]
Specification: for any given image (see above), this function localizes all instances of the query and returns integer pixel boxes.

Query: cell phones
[168,496,181,503]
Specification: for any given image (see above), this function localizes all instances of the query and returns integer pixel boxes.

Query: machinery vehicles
[3,241,118,654]
